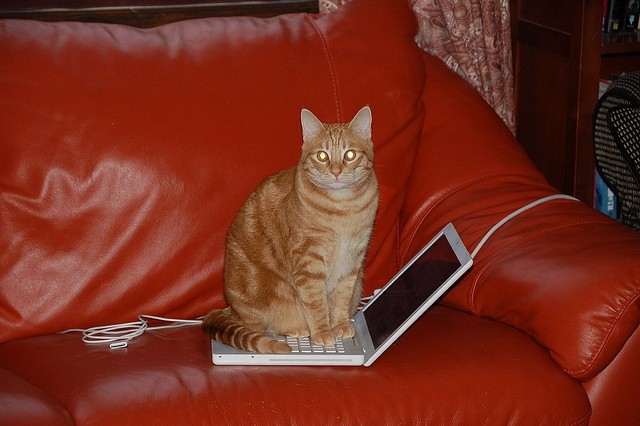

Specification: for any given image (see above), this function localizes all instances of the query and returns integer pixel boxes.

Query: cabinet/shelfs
[509,0,639,227]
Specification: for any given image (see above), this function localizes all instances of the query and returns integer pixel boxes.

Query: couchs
[0,0,635,426]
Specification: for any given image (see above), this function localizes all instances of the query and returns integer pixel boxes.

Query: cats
[201,105,379,353]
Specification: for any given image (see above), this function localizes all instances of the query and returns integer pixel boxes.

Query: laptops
[210,221,474,368]
